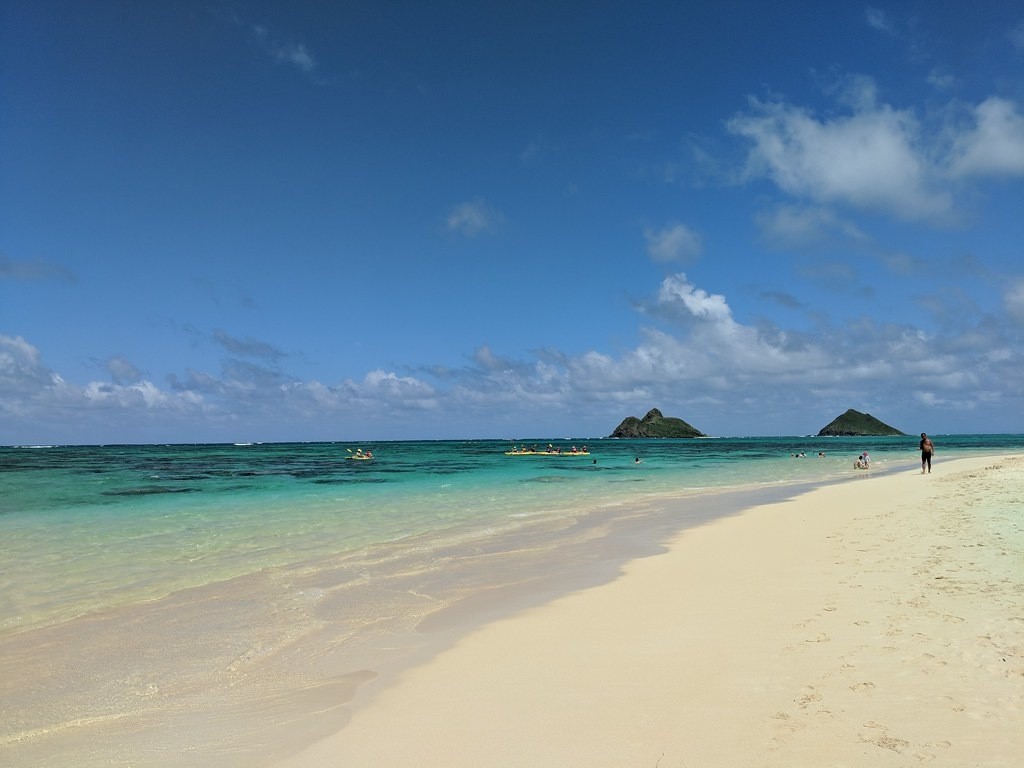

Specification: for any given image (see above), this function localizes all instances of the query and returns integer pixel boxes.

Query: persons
[790,452,825,458]
[854,452,871,469]
[917,432,934,474]
[511,444,587,453]
[356,449,372,458]
[593,451,730,464]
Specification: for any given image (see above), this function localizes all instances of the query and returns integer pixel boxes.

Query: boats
[504,451,591,457]
[345,455,375,460]
